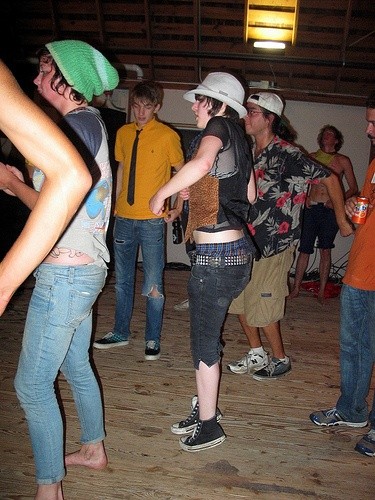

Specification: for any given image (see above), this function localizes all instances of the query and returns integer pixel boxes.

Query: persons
[285,126,359,306]
[148,72,256,453]
[225,92,355,382]
[309,97,375,457]
[0,40,119,500]
[0,61,92,317]
[92,80,185,359]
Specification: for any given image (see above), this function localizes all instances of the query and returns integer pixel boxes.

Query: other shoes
[173,298,190,309]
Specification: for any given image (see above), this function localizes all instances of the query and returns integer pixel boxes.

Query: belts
[195,254,250,265]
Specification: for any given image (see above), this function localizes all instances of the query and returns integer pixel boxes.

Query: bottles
[172,217,182,244]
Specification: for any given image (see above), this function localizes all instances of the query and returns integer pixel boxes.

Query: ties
[127,129,142,206]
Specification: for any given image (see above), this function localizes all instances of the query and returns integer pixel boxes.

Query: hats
[46,40,120,102]
[247,92,284,117]
[183,72,247,119]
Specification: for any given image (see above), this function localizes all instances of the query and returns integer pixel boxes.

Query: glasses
[247,110,272,117]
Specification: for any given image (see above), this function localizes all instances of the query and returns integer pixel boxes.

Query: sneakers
[310,407,367,427]
[253,356,291,381]
[179,416,226,452]
[145,340,161,360]
[356,429,375,456]
[171,400,223,435]
[227,351,271,374]
[93,331,129,349]
